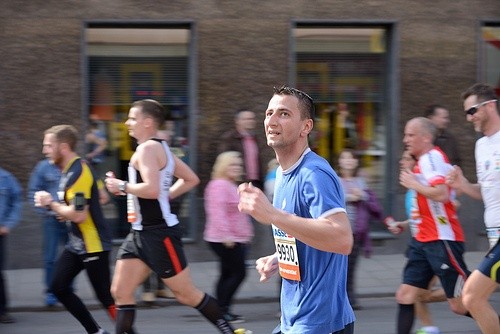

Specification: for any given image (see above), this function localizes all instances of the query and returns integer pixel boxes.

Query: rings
[249,182,255,191]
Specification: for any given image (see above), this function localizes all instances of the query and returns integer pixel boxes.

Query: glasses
[466,99,498,115]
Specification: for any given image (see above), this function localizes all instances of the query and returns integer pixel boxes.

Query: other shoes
[141,291,157,301]
[234,327,252,334]
[156,289,175,298]
[0,312,16,322]
[223,313,244,323]
[47,301,64,308]
[349,300,361,308]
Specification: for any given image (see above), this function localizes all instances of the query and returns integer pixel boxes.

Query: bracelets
[46,199,56,210]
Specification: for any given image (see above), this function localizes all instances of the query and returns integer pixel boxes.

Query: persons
[217,109,269,268]
[26,159,69,308]
[332,149,384,310]
[384,102,479,334]
[103,98,236,334]
[0,167,23,323]
[238,87,354,334]
[35,124,136,334]
[202,152,255,323]
[80,115,187,303]
[461,83,500,334]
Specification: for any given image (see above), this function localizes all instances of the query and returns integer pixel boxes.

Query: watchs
[119,181,129,195]
